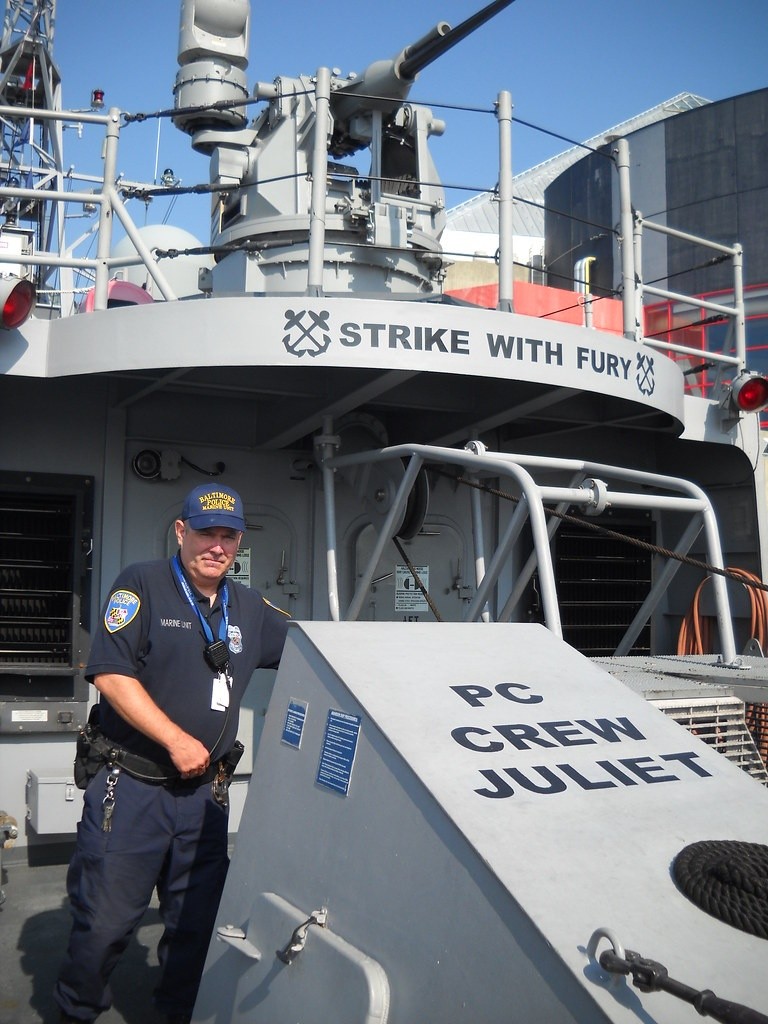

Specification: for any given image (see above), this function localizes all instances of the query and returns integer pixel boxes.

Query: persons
[51,482,291,1024]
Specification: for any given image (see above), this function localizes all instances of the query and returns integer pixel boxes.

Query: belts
[108,749,217,786]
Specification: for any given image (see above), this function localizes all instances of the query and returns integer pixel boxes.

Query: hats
[181,483,247,534]
